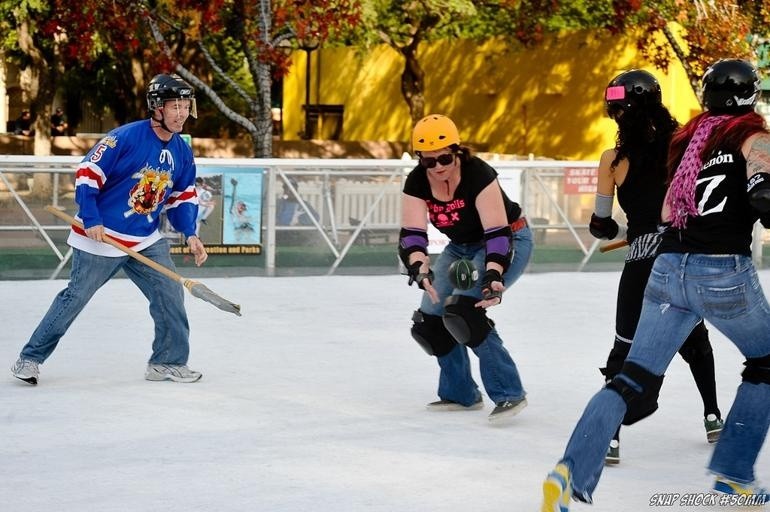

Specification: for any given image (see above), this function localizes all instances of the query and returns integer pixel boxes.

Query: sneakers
[10,356,39,384]
[541,460,573,512]
[488,398,528,420]
[145,363,202,383]
[605,439,619,464]
[711,476,770,503]
[704,413,725,442]
[427,392,483,410]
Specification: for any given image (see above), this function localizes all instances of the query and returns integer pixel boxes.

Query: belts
[511,216,528,231]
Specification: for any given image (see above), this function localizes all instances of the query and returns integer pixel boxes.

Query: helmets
[412,114,461,154]
[145,73,198,120]
[703,58,763,115]
[605,69,661,118]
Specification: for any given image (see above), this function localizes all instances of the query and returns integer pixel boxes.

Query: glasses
[418,153,455,168]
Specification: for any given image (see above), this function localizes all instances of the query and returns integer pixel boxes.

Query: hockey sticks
[44,204,242,317]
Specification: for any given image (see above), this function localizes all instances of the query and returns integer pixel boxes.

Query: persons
[397,114,533,422]
[229,178,256,245]
[9,73,208,385]
[276,178,321,247]
[540,60,770,512]
[195,177,215,239]
[588,70,725,463]
